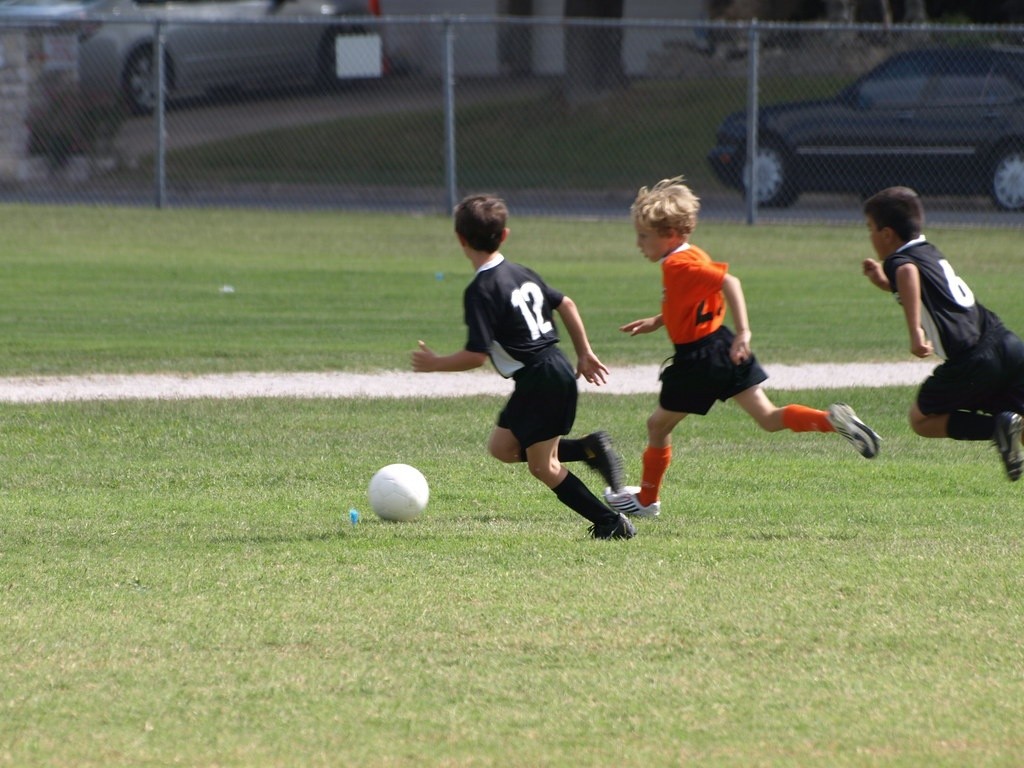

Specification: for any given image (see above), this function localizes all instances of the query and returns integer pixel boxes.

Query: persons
[864,185,1024,482]
[605,175,882,519]
[410,194,635,538]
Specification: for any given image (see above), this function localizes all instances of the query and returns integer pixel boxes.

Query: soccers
[368,462,429,522]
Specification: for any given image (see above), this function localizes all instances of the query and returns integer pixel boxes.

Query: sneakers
[583,512,638,541]
[828,401,882,460]
[606,493,662,518]
[580,430,625,495]
[993,411,1024,482]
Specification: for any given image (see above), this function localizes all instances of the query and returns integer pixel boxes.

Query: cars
[73,1,388,119]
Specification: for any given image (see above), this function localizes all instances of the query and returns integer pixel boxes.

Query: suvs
[705,43,1024,215]
